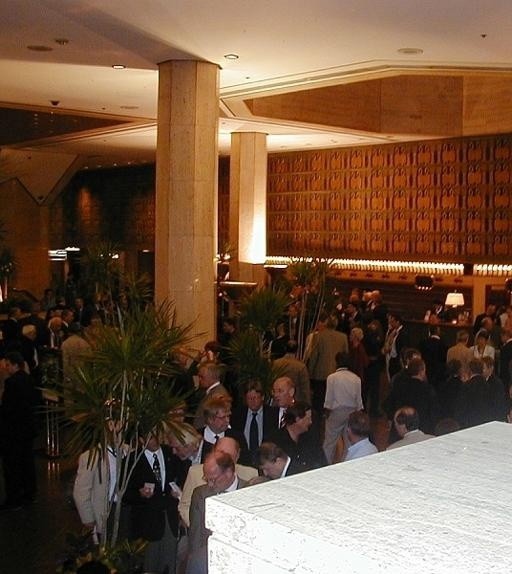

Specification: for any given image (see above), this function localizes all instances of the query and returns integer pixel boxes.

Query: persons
[1,285,118,406]
[75,291,512,574]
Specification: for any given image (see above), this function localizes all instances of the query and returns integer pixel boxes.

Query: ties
[152,453,162,488]
[280,410,287,429]
[250,412,258,451]
[214,434,219,445]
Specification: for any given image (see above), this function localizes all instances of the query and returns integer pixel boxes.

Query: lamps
[415,276,434,290]
[445,293,465,324]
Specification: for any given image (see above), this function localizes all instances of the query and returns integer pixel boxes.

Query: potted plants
[36,239,209,574]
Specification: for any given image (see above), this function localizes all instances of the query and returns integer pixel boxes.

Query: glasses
[202,475,220,484]
